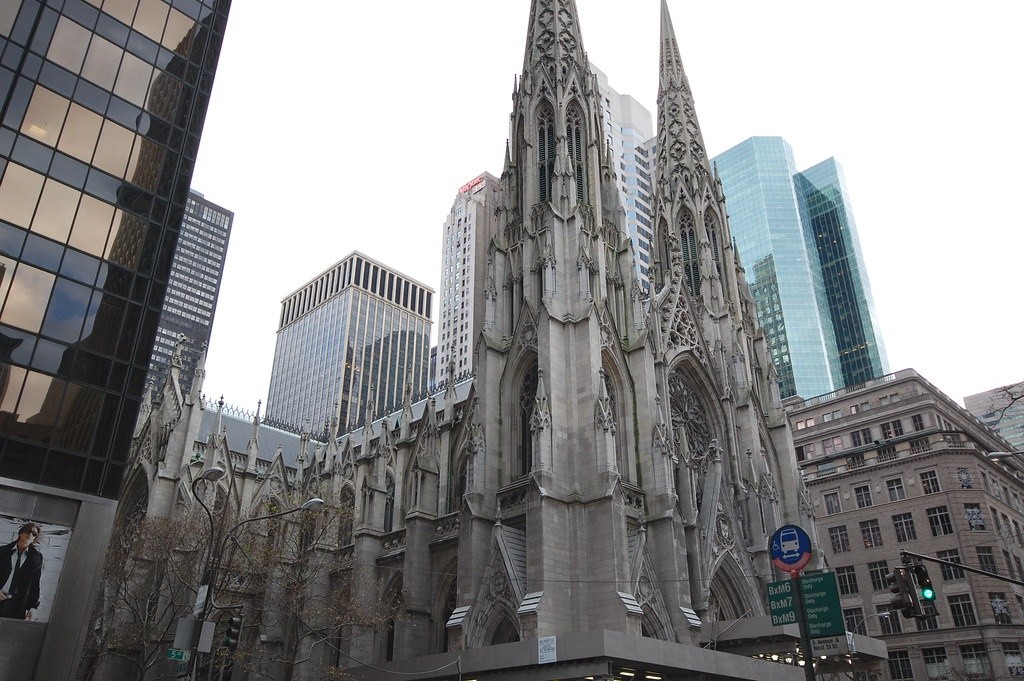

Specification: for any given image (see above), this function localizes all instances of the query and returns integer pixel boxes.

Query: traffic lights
[885,567,918,618]
[222,614,245,652]
[913,565,938,603]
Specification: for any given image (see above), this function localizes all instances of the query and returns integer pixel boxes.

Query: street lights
[182,464,326,680]
[848,612,891,680]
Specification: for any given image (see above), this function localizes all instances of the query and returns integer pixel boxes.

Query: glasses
[24,527,38,537]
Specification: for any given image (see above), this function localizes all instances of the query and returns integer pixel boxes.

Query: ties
[9,542,27,595]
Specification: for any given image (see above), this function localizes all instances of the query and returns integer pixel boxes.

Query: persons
[0,523,43,621]
